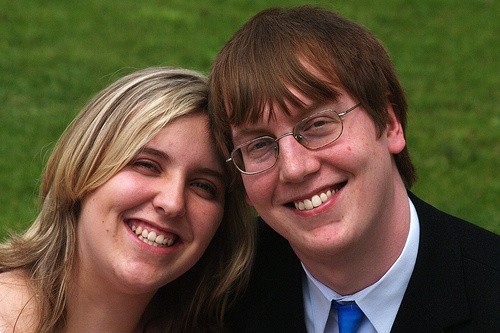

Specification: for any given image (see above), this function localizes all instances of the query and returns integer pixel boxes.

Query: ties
[332,300,365,333]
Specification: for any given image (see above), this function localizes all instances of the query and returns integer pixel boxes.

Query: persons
[207,7,500,332]
[0,66,258,333]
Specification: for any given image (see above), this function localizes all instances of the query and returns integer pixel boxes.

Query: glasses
[226,102,363,175]
